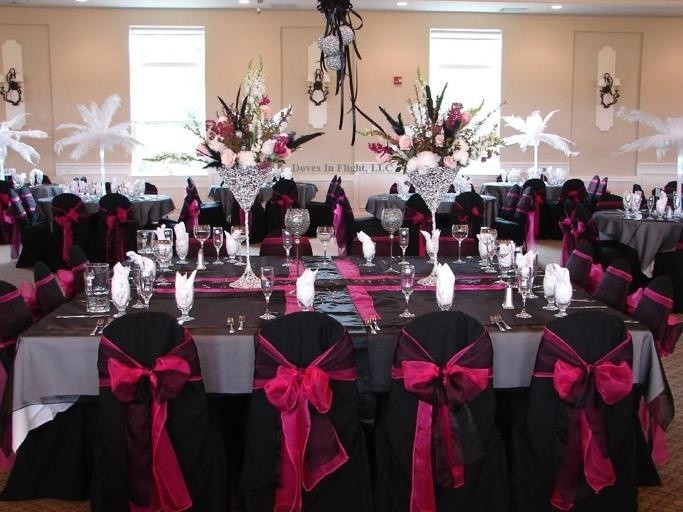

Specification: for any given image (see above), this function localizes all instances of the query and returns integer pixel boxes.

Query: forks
[237,315,247,331]
[90,317,114,336]
[364,312,382,335]
[226,317,235,335]
[489,314,514,334]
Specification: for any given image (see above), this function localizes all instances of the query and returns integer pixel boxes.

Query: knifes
[55,313,111,319]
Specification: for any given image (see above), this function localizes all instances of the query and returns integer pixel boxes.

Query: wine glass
[5,168,46,189]
[622,190,681,223]
[110,279,131,318]
[58,179,146,203]
[175,284,196,321]
[279,207,335,296]
[133,263,153,313]
[176,225,248,265]
[392,173,473,199]
[425,223,569,319]
[399,265,418,318]
[153,239,172,286]
[259,265,277,321]
[360,208,412,275]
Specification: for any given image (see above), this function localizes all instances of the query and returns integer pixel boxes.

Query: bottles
[163,229,173,240]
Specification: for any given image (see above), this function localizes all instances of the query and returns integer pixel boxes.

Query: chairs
[1,172,683,511]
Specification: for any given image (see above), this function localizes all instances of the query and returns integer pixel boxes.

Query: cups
[136,230,155,258]
[296,282,315,311]
[499,168,565,186]
[83,263,110,313]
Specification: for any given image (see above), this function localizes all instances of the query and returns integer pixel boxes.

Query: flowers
[141,54,325,172]
[345,63,509,176]
